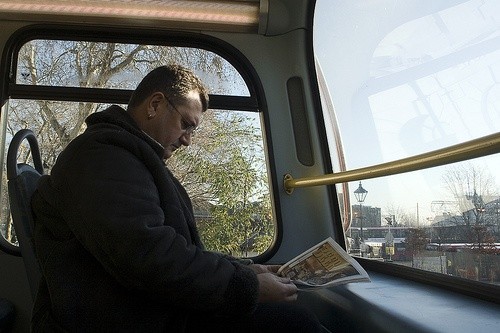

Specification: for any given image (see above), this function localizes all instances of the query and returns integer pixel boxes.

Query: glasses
[165,96,194,134]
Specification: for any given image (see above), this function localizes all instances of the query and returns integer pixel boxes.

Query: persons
[31,64,299,333]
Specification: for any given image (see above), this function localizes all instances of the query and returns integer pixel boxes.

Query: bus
[345,226,418,248]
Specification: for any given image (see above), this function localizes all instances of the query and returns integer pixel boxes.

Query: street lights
[437,236,443,273]
[353,181,369,258]
[385,215,395,262]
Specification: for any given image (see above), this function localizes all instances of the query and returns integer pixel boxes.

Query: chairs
[6,129,147,333]
